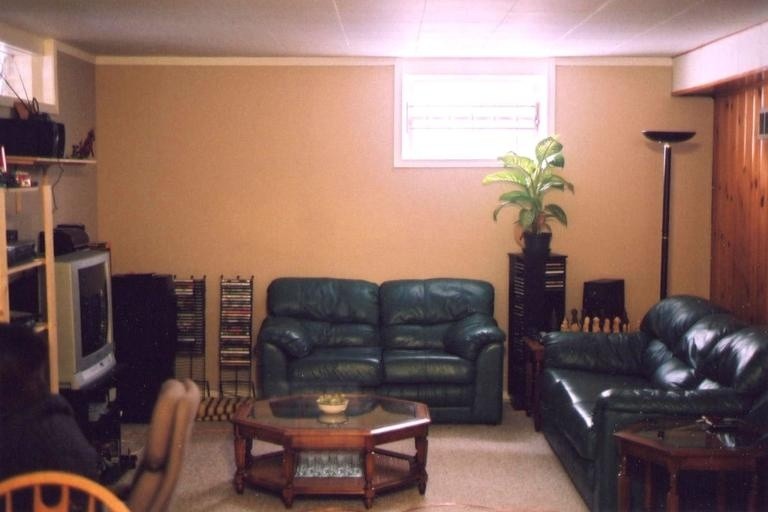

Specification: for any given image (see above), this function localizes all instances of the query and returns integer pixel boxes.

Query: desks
[611,416,768,511]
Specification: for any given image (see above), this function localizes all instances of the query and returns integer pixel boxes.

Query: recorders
[0,111,66,159]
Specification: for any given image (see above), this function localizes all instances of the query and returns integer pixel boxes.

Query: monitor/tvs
[39,246,118,391]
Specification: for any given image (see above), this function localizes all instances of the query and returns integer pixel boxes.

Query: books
[174,280,204,354]
[510,262,564,375]
[221,278,251,366]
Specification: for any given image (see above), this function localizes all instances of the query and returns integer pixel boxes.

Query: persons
[1,323,132,512]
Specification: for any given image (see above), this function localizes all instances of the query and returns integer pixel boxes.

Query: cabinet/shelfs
[0,179,58,397]
[507,251,569,415]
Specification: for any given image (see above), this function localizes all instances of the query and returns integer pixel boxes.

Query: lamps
[644,130,698,300]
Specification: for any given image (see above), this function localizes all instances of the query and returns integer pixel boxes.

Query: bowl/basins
[316,397,349,414]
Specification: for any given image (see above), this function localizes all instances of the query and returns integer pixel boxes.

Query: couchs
[252,274,508,426]
[538,293,768,511]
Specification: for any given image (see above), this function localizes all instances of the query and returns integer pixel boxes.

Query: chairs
[101,377,200,512]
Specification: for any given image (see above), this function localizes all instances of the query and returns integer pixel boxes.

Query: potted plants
[483,132,576,256]
